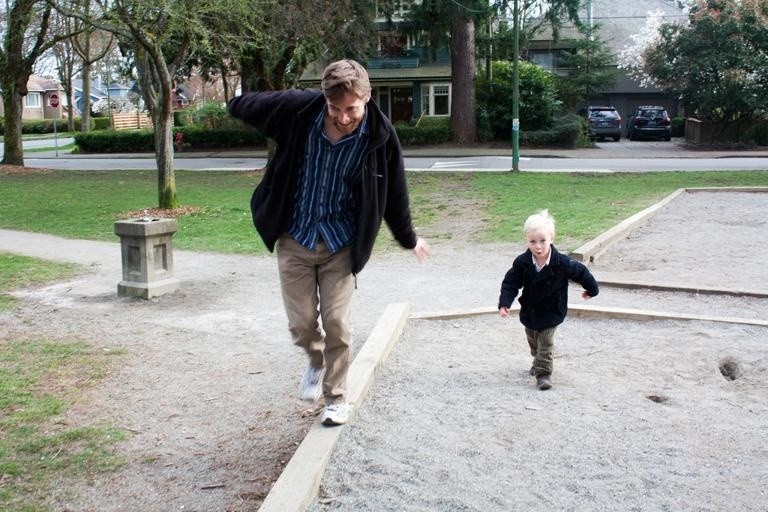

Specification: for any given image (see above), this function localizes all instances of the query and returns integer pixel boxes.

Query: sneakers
[298,362,326,404]
[321,394,349,427]
[537,371,552,390]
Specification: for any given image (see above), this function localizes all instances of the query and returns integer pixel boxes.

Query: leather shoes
[530,364,538,376]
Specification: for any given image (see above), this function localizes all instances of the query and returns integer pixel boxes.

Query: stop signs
[49,94,60,108]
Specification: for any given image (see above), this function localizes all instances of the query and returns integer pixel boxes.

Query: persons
[495,208,601,391]
[221,57,431,428]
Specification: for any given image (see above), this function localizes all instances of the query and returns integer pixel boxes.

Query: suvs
[625,103,673,141]
[573,104,624,144]
